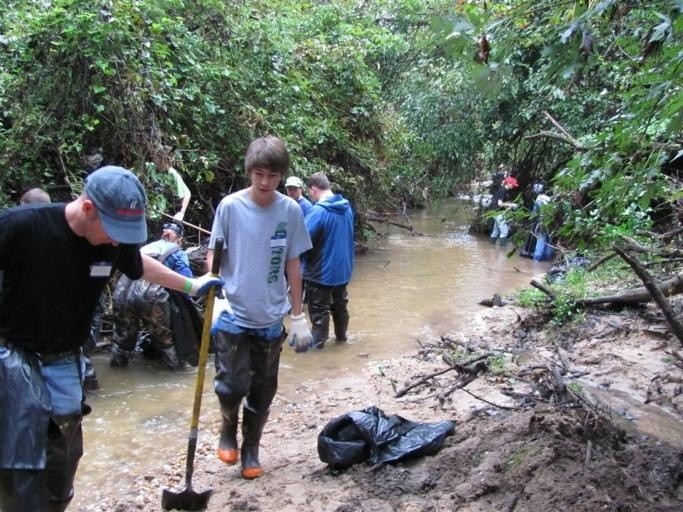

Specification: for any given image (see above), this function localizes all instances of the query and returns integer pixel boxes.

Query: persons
[19,188,50,205]
[204,137,315,479]
[284,176,312,218]
[299,172,354,349]
[128,146,192,240]
[489,177,518,248]
[0,165,225,512]
[529,183,561,263]
[108,221,192,370]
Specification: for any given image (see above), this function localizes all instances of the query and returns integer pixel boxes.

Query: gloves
[183,274,225,302]
[289,315,315,352]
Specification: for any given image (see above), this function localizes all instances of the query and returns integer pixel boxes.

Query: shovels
[161,237,224,512]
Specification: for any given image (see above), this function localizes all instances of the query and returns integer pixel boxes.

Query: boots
[217,401,240,464]
[498,237,505,245]
[489,237,497,244]
[241,404,269,478]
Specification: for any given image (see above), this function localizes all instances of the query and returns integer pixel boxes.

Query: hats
[88,165,149,245]
[506,177,518,186]
[285,176,303,187]
